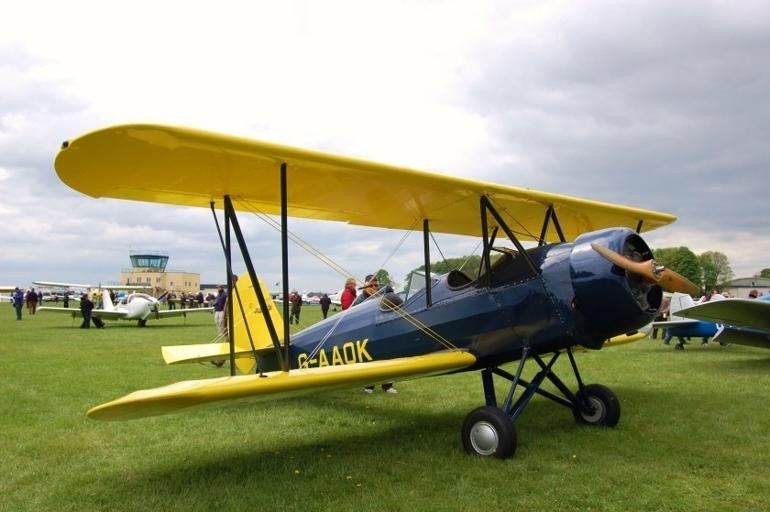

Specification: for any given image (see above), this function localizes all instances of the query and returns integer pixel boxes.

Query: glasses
[369,284,378,287]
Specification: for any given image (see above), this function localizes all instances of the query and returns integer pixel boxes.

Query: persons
[80,293,93,331]
[321,293,332,320]
[12,286,44,321]
[63,292,71,310]
[647,286,770,349]
[341,277,357,311]
[289,291,303,325]
[213,285,228,338]
[356,274,398,397]
[213,275,238,366]
[167,286,216,310]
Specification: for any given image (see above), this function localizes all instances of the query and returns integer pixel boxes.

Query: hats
[365,274,379,283]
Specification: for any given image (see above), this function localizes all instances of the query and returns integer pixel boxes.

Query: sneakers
[364,389,373,394]
[387,388,397,394]
